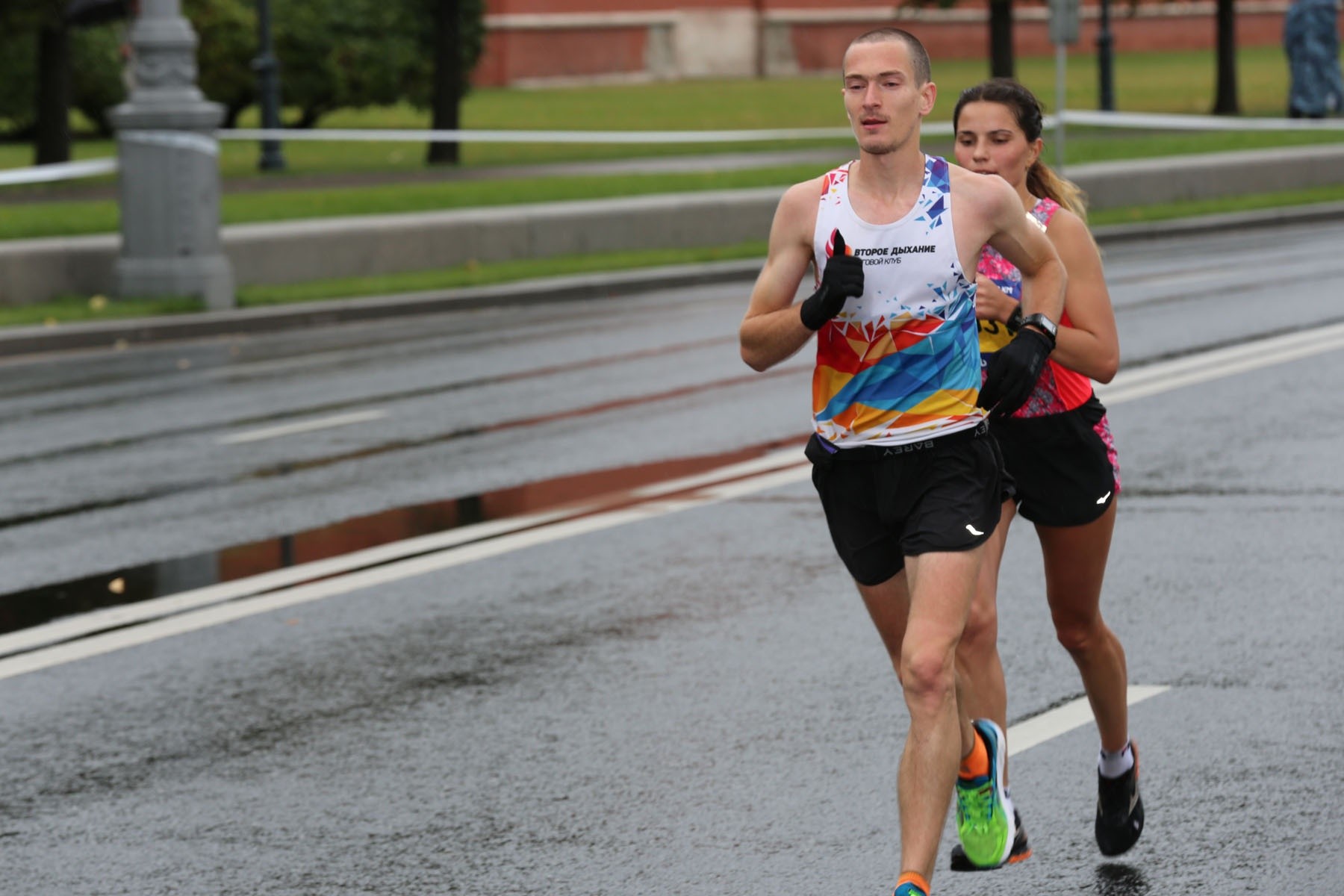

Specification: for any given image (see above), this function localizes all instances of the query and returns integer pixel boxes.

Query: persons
[953,77,1145,866]
[1279,0,1343,120]
[740,27,1016,896]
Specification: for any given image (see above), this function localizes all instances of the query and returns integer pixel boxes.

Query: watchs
[1006,304,1023,333]
[1019,313,1058,343]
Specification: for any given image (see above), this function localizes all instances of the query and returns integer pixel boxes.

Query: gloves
[799,229,866,332]
[975,328,1055,424]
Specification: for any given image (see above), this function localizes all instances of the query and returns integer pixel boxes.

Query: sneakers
[956,717,1016,868]
[951,823,1031,871]
[895,882,927,896]
[1094,741,1144,857]
